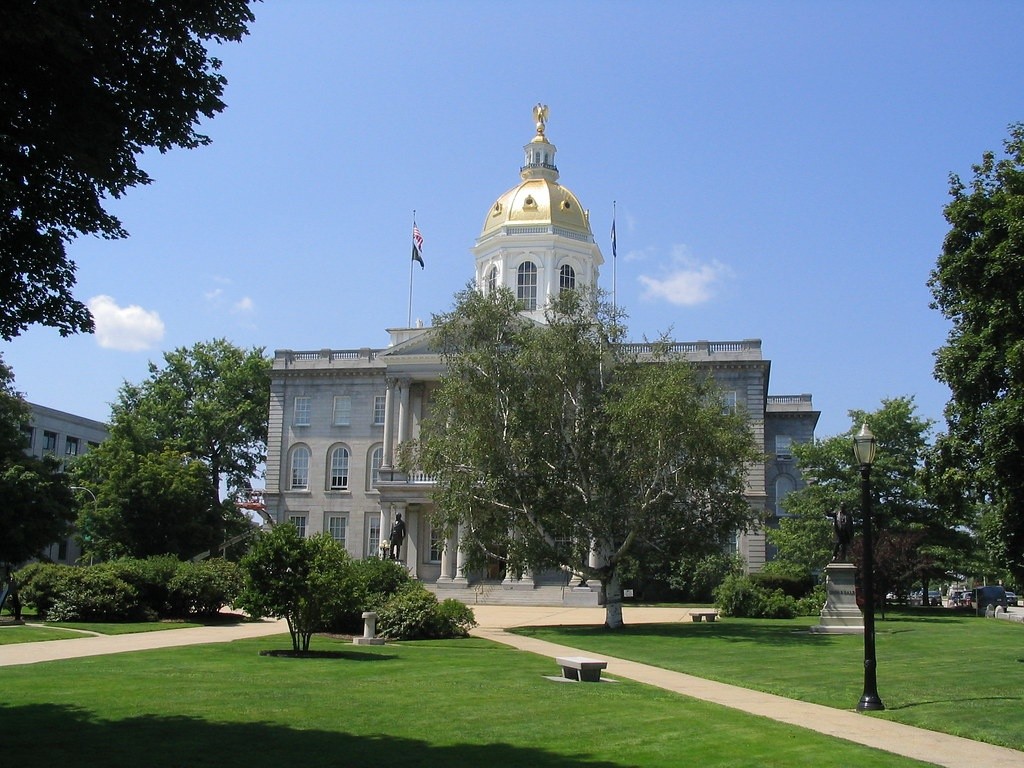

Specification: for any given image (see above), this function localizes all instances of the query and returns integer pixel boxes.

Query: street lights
[71,487,97,566]
[852,424,886,710]
[380,540,389,559]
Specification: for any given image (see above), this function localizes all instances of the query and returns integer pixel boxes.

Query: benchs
[689,612,718,622]
[556,656,607,682]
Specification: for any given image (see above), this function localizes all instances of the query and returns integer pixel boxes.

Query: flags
[610,208,617,258]
[411,220,425,270]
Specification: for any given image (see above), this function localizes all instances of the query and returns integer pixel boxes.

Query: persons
[824,502,854,563]
[389,512,406,559]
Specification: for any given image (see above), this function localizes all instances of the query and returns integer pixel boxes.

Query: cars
[928,591,942,605]
[946,586,1018,611]
